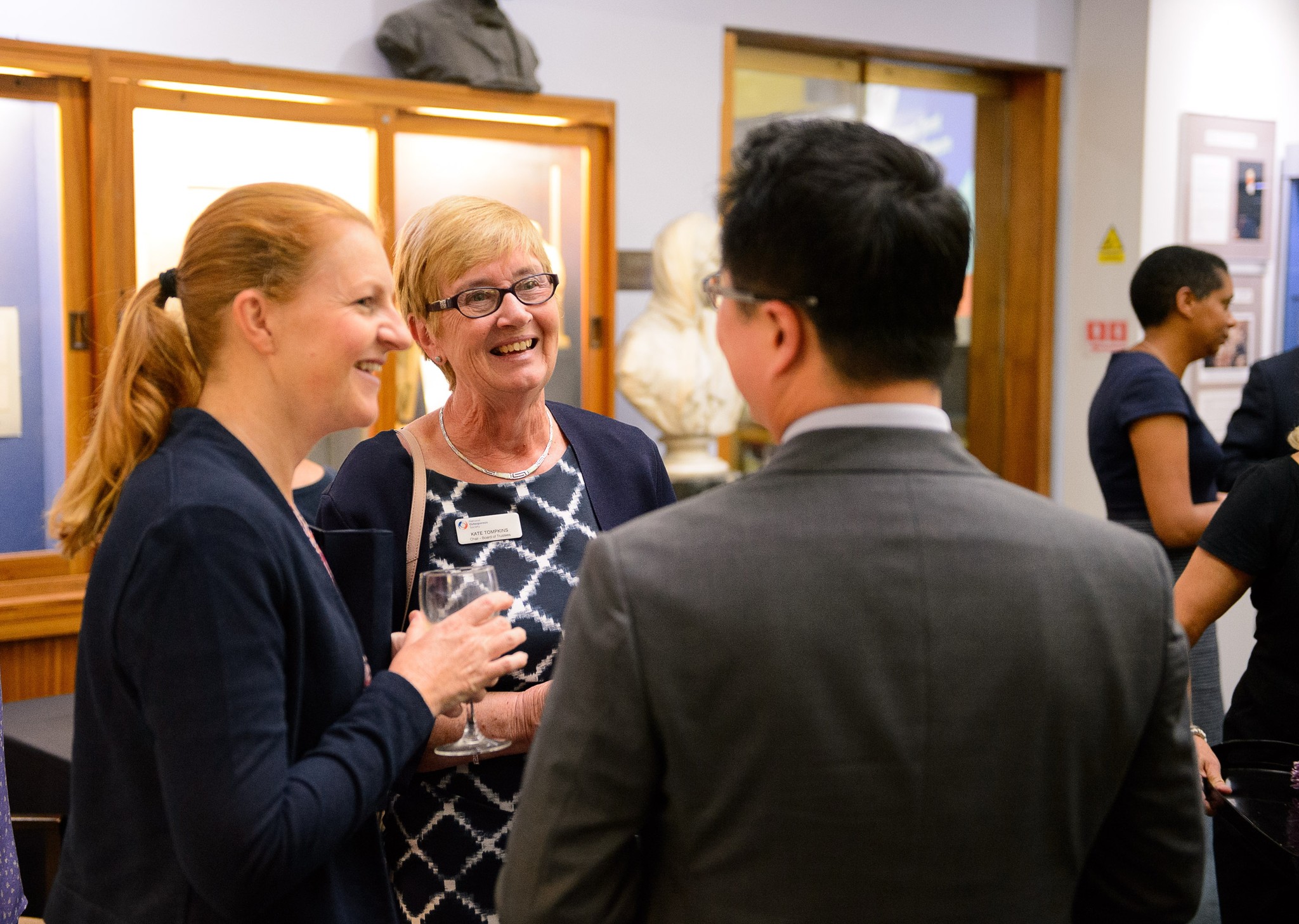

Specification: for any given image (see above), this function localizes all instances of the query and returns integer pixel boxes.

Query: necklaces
[438,406,553,479]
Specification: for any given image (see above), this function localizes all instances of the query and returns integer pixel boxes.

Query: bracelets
[1190,725,1207,742]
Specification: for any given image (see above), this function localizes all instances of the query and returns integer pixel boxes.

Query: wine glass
[416,564,515,756]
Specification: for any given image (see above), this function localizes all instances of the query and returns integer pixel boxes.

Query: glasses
[424,273,559,319]
[701,272,817,309]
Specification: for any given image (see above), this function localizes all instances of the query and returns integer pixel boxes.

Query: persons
[614,213,745,438]
[50,182,528,924]
[495,119,1207,924]
[314,196,674,923]
[1088,244,1299,924]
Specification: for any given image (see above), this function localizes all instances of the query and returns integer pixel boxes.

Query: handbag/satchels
[1202,740,1299,890]
